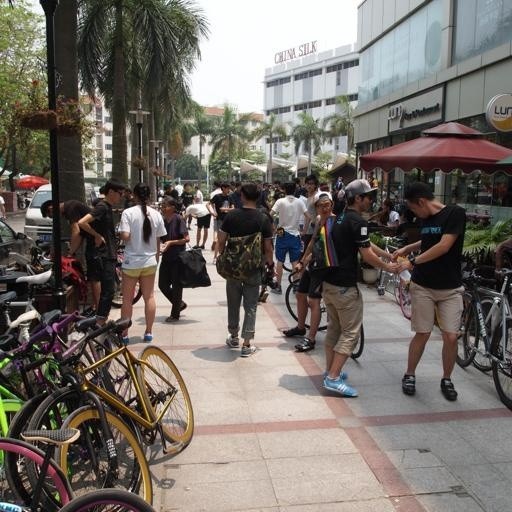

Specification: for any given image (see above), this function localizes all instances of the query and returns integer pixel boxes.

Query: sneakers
[181,301,187,310]
[80,308,97,317]
[324,370,347,380]
[226,334,240,347]
[166,315,178,322]
[123,336,130,345]
[323,377,360,397]
[144,332,153,342]
[295,338,315,351]
[261,290,269,302]
[242,344,257,356]
[271,285,284,295]
[403,373,417,393]
[442,377,458,400]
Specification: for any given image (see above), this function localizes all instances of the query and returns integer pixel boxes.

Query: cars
[0,219,40,278]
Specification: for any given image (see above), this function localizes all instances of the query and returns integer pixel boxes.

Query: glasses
[315,203,331,208]
[117,190,125,197]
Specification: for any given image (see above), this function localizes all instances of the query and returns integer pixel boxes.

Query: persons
[76,178,124,328]
[367,170,378,190]
[215,183,273,357]
[182,198,211,249]
[395,208,416,235]
[334,176,344,190]
[382,199,399,230]
[193,186,202,204]
[209,180,224,251]
[162,184,177,200]
[394,181,466,399]
[280,190,336,351]
[319,179,398,397]
[409,258,416,266]
[302,174,320,256]
[123,187,135,209]
[174,180,183,198]
[204,182,234,255]
[0,195,7,226]
[117,182,167,346]
[158,196,190,323]
[268,181,312,295]
[40,199,102,319]
[262,178,307,204]
[181,182,195,231]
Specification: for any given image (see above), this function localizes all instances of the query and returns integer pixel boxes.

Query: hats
[313,192,333,205]
[344,180,378,199]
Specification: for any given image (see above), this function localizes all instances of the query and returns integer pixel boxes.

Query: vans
[23,183,98,251]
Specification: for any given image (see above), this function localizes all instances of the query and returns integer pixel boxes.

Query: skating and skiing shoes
[283,326,306,337]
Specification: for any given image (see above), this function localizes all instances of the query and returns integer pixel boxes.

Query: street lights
[148,139,163,202]
[127,85,151,185]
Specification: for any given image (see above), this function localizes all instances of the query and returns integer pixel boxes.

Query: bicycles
[0,231,194,512]
[456,267,511,409]
[176,199,185,216]
[378,236,417,320]
[281,262,365,359]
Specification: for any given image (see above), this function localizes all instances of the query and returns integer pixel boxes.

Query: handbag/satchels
[217,214,266,285]
[311,216,338,271]
[176,236,212,287]
